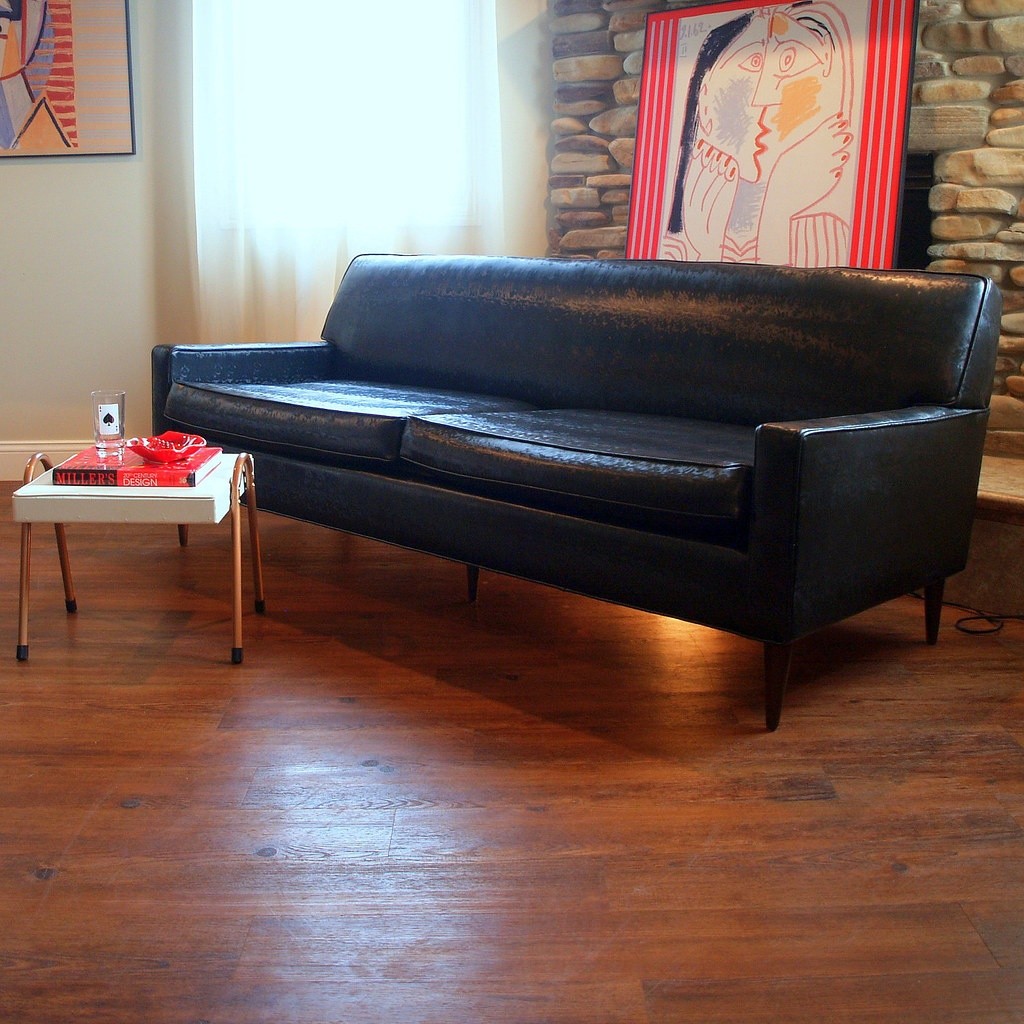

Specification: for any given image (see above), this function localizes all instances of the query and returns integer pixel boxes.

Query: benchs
[151,249,997,735]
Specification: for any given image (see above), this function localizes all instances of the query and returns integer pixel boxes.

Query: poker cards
[98,404,120,435]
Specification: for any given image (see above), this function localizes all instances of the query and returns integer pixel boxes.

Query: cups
[91,390,125,448]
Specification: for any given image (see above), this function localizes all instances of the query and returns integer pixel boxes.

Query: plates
[126,431,206,462]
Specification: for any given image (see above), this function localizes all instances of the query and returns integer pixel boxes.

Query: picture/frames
[0,0,137,158]
[624,0,920,270]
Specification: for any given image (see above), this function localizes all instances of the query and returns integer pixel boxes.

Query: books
[52,445,223,487]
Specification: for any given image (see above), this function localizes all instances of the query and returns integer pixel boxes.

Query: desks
[11,442,267,667]
